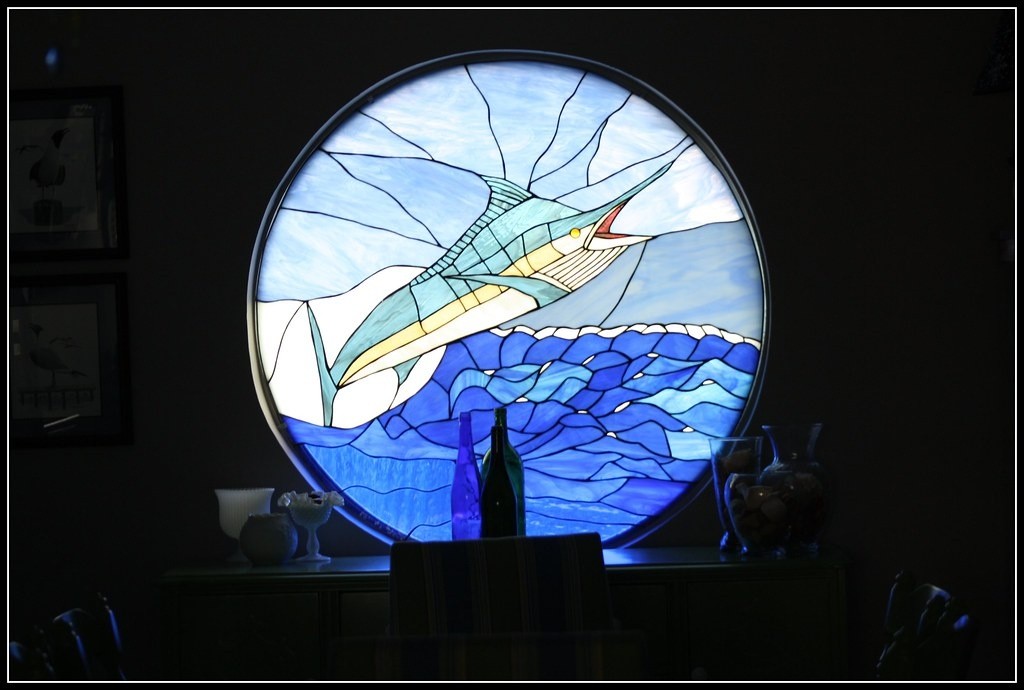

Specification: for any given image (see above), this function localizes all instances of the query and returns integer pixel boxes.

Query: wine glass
[283,489,347,565]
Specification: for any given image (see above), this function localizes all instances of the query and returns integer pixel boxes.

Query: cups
[724,467,796,561]
[706,435,766,553]
[214,487,276,561]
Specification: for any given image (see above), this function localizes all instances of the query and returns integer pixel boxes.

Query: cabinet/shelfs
[155,547,788,681]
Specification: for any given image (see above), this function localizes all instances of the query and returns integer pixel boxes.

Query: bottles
[480,425,519,538]
[240,514,297,567]
[762,422,832,563]
[449,409,480,541]
[483,406,527,538]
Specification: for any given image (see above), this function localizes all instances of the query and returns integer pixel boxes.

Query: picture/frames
[9,89,130,258]
[11,278,132,445]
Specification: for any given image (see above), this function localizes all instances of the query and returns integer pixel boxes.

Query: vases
[724,476,786,563]
[763,424,828,556]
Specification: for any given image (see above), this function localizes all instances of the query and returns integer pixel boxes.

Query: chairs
[881,571,975,680]
[9,595,125,679]
[376,534,642,679]
